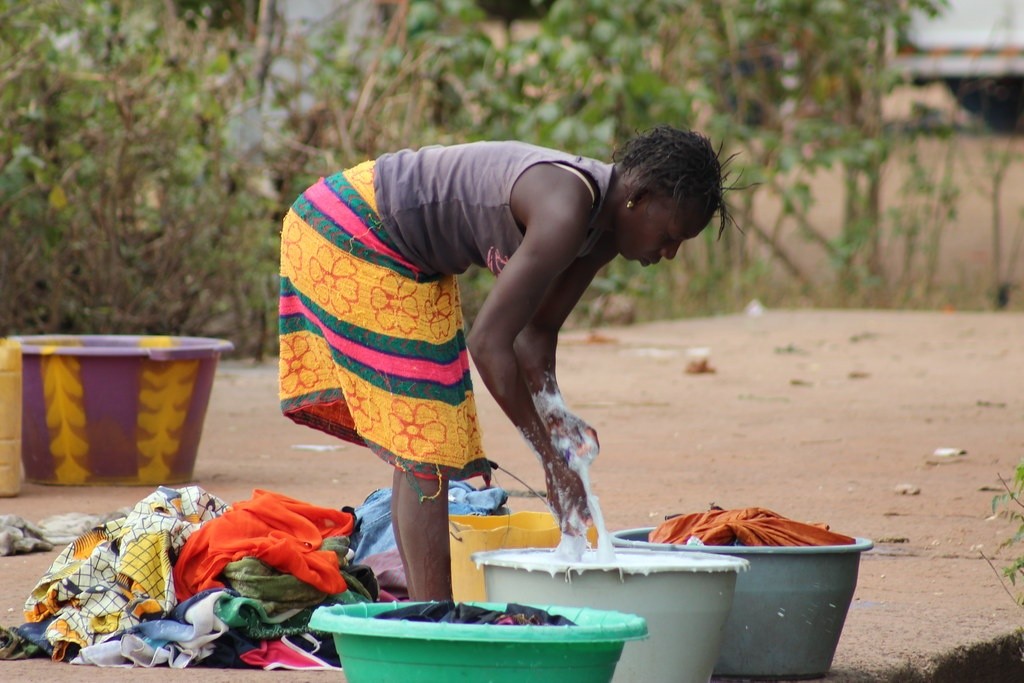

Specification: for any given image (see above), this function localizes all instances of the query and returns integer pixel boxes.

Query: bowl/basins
[609,526,874,680]
[8,333,236,487]
[307,601,650,683]
[471,546,750,683]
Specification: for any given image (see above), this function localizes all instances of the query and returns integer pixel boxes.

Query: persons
[278,123,764,602]
